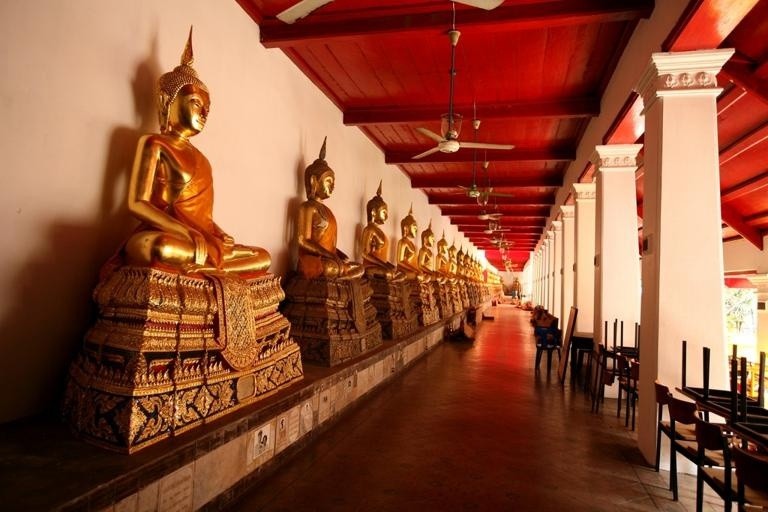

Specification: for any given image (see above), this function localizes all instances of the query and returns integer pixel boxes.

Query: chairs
[535,328,562,373]
[570,319,768,512]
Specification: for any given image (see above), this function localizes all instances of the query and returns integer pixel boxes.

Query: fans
[275,0,506,25]
[410,3,515,160]
[454,97,517,273]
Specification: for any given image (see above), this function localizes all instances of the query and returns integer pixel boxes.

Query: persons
[121,24,272,276]
[358,179,408,283]
[396,203,484,284]
[292,136,366,282]
